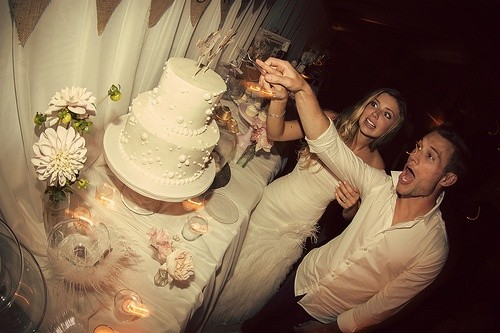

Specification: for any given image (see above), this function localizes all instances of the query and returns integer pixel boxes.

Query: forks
[235,42,276,91]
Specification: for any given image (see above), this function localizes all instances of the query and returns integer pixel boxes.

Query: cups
[181,211,208,242]
[95,182,115,204]
[0,206,152,333]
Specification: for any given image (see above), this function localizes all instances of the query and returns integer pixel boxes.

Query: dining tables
[78,96,287,333]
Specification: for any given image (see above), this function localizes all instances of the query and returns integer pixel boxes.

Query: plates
[239,101,267,130]
[203,192,240,225]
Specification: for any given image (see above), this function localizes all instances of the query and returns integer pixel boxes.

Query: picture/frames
[260,29,291,53]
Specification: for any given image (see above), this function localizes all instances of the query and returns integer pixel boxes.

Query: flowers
[250,128,275,152]
[31,84,123,200]
[146,227,194,281]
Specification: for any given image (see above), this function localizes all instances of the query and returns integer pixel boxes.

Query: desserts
[237,92,270,122]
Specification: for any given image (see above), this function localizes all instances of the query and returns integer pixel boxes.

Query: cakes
[119,56,227,186]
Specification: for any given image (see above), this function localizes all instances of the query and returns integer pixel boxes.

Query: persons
[187,59,408,333]
[241,57,476,333]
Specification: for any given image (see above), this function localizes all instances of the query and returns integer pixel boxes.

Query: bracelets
[266,109,286,118]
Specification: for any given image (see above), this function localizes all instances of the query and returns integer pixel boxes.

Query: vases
[41,187,69,239]
